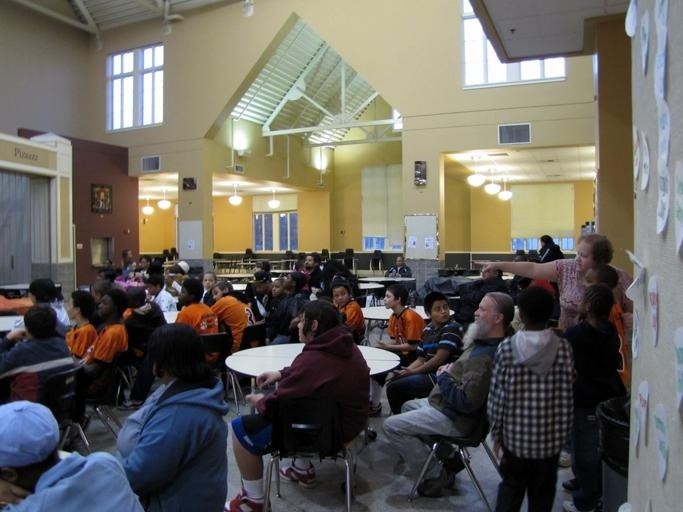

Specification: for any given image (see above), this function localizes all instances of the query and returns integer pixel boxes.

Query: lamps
[229,183,243,206]
[268,189,281,209]
[142,198,154,215]
[157,189,171,209]
[467,155,513,202]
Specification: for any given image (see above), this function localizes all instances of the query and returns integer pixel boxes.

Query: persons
[371,256,516,496]
[1,246,369,512]
[475,234,636,512]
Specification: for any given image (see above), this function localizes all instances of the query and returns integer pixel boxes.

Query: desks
[213,260,242,274]
[268,261,282,271]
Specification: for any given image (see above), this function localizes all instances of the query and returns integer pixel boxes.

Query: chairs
[243,248,255,272]
[161,248,177,261]
[513,249,541,263]
[407,395,502,512]
[282,247,384,271]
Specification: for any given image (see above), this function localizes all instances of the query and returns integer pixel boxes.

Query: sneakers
[367,400,382,417]
[558,451,597,512]
[223,487,271,512]
[417,451,470,497]
[117,400,144,410]
[279,458,317,487]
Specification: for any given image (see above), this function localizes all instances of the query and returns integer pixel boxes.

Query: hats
[330,271,351,297]
[0,400,60,467]
